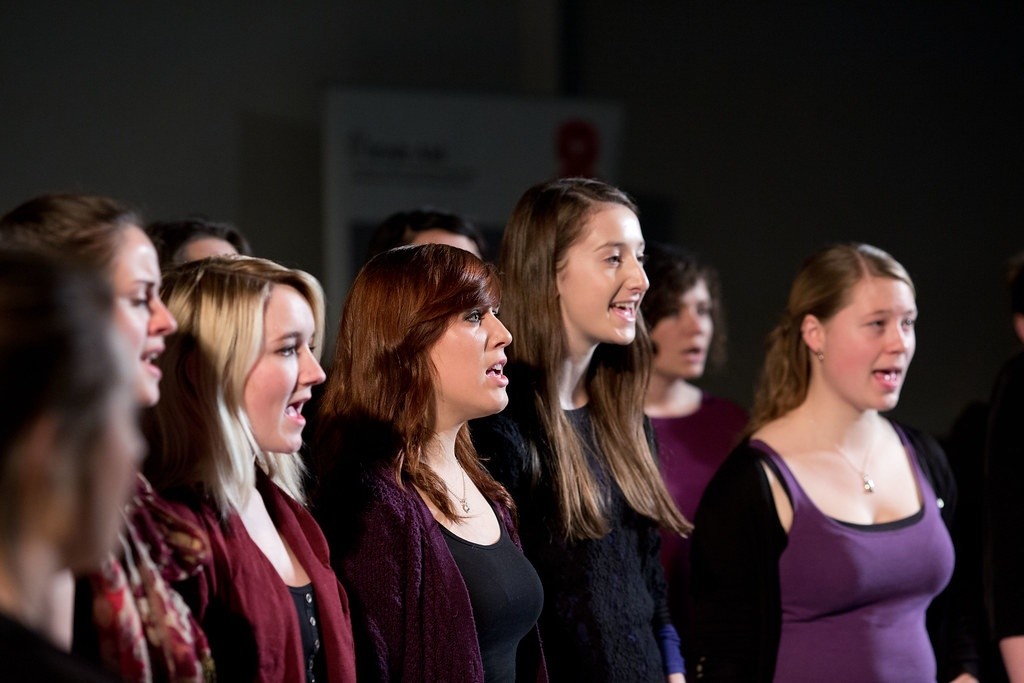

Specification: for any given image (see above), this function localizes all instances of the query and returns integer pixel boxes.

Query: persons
[366,210,489,262]
[141,255,356,683]
[689,234,991,683]
[305,245,550,683]
[0,244,148,683]
[981,252,1024,683]
[0,194,216,683]
[145,220,250,269]
[466,176,684,683]
[639,247,751,574]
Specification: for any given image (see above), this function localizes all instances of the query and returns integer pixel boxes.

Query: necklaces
[801,407,875,494]
[447,459,469,513]
